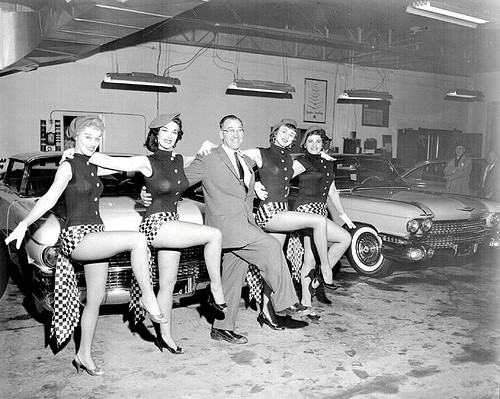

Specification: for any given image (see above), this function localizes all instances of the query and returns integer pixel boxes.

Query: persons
[196,118,337,331]
[58,113,228,354]
[477,147,498,200]
[255,125,356,320]
[442,141,472,195]
[5,114,168,376]
[140,114,313,345]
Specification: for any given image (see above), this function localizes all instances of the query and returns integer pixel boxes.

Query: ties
[234,152,248,193]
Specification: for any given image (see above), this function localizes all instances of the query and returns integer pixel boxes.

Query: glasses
[223,128,243,135]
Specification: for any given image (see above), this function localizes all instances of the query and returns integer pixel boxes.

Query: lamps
[227,31,294,94]
[447,89,484,100]
[339,51,394,101]
[405,0,492,28]
[104,39,180,87]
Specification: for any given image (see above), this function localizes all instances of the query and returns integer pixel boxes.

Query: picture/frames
[302,77,328,123]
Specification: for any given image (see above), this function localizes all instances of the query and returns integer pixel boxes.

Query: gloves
[254,181,268,200]
[340,212,356,229]
[4,220,28,251]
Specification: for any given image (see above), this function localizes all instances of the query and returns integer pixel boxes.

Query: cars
[192,153,500,279]
[0,150,205,317]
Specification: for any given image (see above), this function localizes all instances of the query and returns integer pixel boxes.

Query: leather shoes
[275,301,314,317]
[211,326,248,344]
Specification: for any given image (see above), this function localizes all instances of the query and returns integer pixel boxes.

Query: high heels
[74,353,105,376]
[308,312,320,321]
[305,268,320,296]
[139,297,168,324]
[205,283,228,312]
[313,264,338,290]
[156,336,184,355]
[259,311,285,330]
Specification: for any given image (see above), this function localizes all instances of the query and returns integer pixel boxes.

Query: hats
[300,125,322,147]
[270,118,298,134]
[148,112,181,129]
